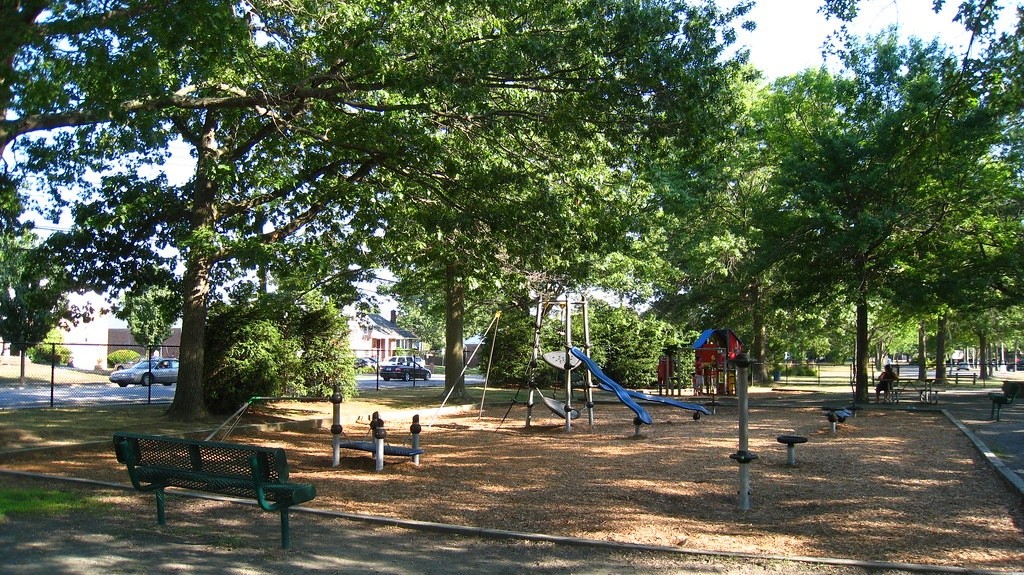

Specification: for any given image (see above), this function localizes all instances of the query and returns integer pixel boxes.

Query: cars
[110,357,179,387]
[113,356,142,371]
[956,362,969,371]
[353,356,378,369]
[1006,360,1024,372]
[379,361,432,382]
[381,356,425,368]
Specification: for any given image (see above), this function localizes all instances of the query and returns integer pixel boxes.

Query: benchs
[112,433,317,549]
[880,379,937,405]
[947,373,977,385]
[875,388,946,407]
[988,381,1018,423]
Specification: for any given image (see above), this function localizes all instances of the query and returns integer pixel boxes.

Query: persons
[873,365,899,405]
[695,356,704,396]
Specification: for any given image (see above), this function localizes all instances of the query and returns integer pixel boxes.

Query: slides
[571,346,653,425]
[597,382,711,416]
